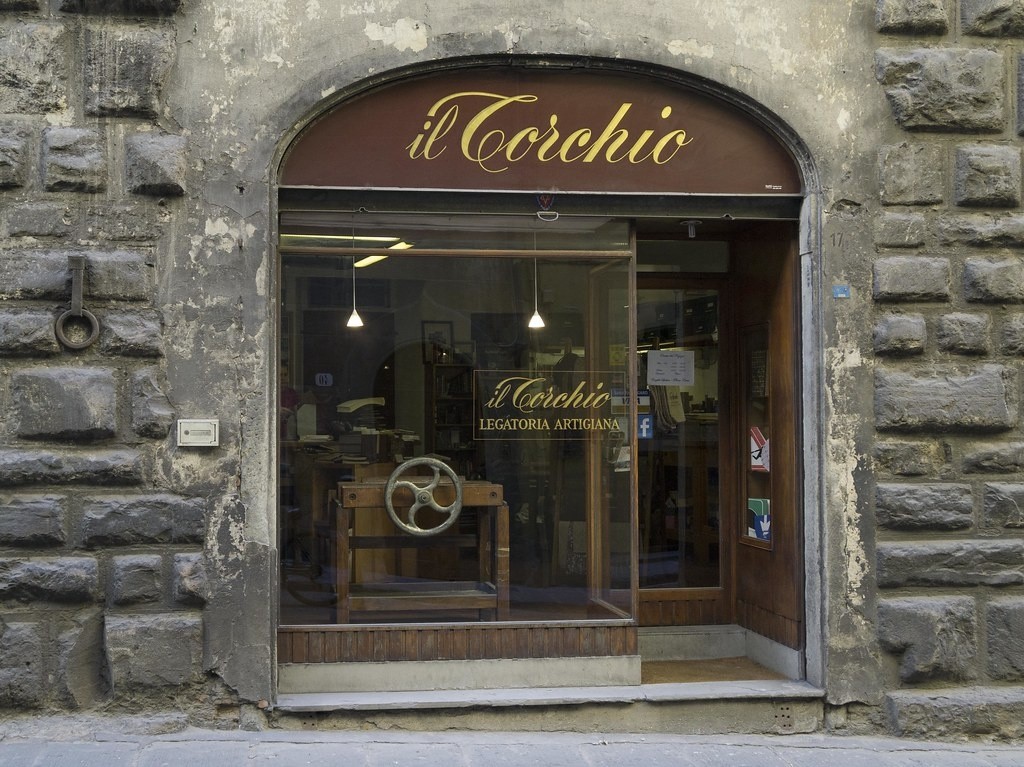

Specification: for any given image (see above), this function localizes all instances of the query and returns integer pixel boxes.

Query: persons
[281,359,303,507]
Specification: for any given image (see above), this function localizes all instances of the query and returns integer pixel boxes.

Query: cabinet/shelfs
[426,364,479,481]
[279,438,461,583]
[280,312,293,390]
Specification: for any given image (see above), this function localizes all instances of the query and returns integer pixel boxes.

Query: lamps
[528,232,545,328]
[347,227,364,327]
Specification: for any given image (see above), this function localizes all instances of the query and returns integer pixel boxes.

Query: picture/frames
[421,321,455,364]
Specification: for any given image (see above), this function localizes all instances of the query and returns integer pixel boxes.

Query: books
[435,402,472,425]
[436,373,474,396]
[459,458,476,480]
[484,346,518,373]
[436,429,475,449]
[685,411,719,425]
[297,396,420,465]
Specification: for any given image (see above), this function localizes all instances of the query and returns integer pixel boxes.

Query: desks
[638,439,719,566]
